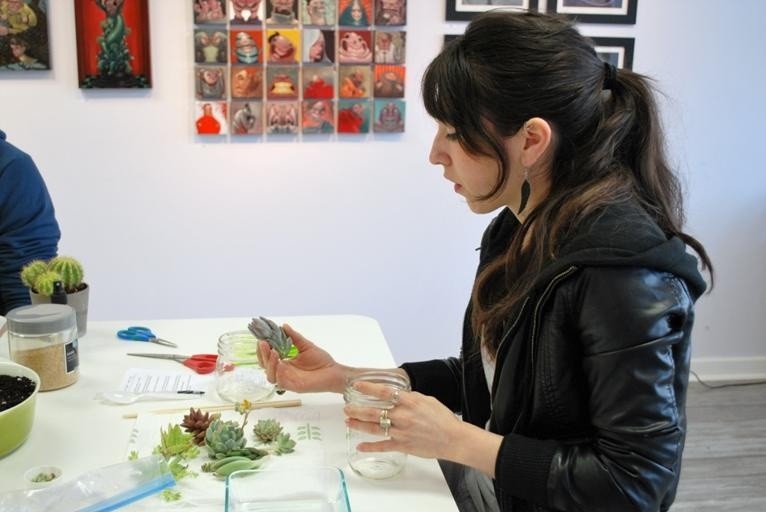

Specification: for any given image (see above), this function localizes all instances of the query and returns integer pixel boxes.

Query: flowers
[204,399,252,457]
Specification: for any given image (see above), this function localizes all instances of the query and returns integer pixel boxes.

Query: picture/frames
[546,0,637,22]
[445,0,537,21]
[443,33,463,51]
[583,36,635,75]
[74,0,152,90]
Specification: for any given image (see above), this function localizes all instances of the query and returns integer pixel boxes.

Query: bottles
[342,368,411,480]
[214,329,276,404]
[6,302,80,392]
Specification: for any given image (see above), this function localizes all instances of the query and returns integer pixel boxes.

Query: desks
[0,316,458,512]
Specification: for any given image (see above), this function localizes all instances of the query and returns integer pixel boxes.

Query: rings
[389,387,401,406]
[379,408,391,431]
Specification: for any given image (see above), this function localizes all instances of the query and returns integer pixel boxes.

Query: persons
[0,131,61,319]
[255,3,715,512]
[9,37,37,63]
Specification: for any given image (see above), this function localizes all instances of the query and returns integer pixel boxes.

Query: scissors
[117,326,177,347]
[127,352,219,374]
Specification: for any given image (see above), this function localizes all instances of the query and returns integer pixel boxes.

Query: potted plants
[20,256,90,336]
